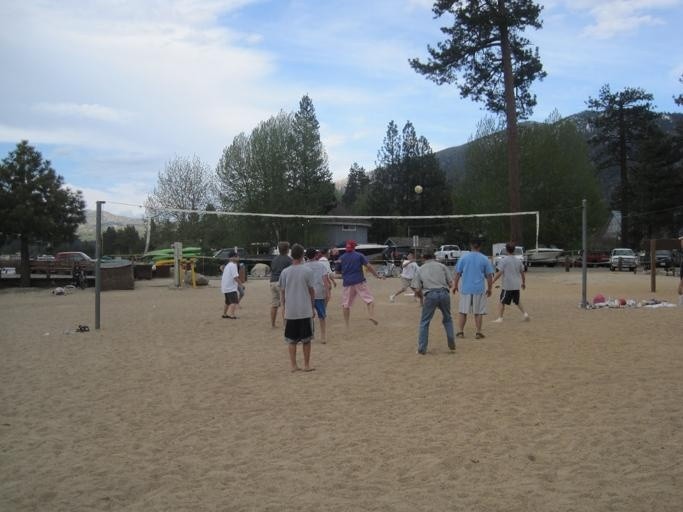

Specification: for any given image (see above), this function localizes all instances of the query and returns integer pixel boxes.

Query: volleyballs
[415,185,423,193]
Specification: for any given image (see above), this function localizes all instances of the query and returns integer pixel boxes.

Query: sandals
[475,333,484,339]
[456,332,463,337]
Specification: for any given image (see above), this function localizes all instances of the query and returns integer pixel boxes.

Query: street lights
[250,241,265,256]
[414,184,425,237]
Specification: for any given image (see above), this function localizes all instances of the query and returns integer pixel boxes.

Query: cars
[654,249,673,268]
[0,249,113,278]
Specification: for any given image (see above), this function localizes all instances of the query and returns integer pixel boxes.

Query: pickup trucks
[205,248,275,275]
[432,244,471,266]
[572,249,608,267]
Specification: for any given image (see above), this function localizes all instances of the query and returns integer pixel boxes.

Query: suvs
[607,247,638,272]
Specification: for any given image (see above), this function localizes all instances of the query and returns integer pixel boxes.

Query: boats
[328,241,388,266]
[524,247,563,266]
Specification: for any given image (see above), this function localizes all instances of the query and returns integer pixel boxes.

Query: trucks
[492,241,526,273]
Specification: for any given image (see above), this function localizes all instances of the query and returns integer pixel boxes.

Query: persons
[452,241,494,339]
[72,262,81,289]
[335,240,382,326]
[565,255,570,272]
[270,240,340,372]
[678,237,683,312]
[410,253,456,355]
[219,245,246,318]
[389,251,424,302]
[493,243,529,322]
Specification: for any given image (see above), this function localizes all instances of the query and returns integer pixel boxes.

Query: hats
[346,240,356,251]
